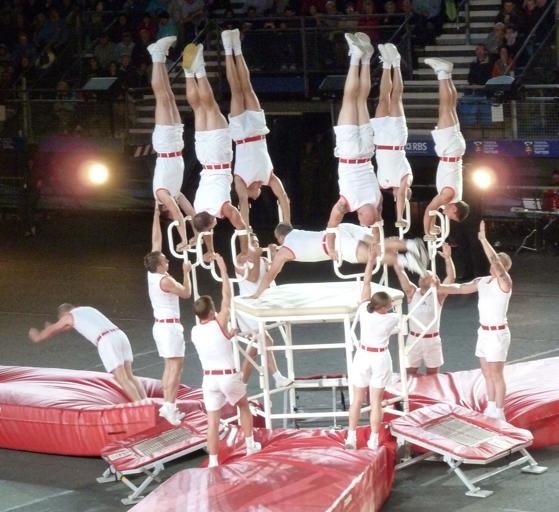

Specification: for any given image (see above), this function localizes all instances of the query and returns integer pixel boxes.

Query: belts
[340,146,403,163]
[155,319,180,323]
[161,152,182,158]
[440,157,459,162]
[482,326,505,330]
[361,346,386,352]
[202,135,265,169]
[97,328,119,342]
[409,331,438,338]
[206,369,237,375]
[324,235,328,255]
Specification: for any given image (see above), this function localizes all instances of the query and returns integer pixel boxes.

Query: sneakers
[276,378,293,388]
[146,29,241,73]
[247,442,261,454]
[424,57,453,73]
[345,32,401,63]
[159,405,185,425]
[345,437,378,448]
[406,237,429,278]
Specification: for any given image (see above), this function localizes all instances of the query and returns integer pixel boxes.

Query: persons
[425,58,466,234]
[222,29,291,233]
[182,44,253,253]
[438,220,514,419]
[191,254,262,469]
[229,234,293,391]
[251,224,429,300]
[21,157,44,238]
[223,2,420,68]
[142,200,192,426]
[370,42,413,228]
[468,1,556,97]
[327,32,383,263]
[146,36,199,249]
[391,241,457,374]
[29,302,147,402]
[345,245,400,448]
[1,1,207,147]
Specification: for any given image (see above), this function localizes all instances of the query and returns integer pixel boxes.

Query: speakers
[82,78,121,103]
[485,75,516,101]
